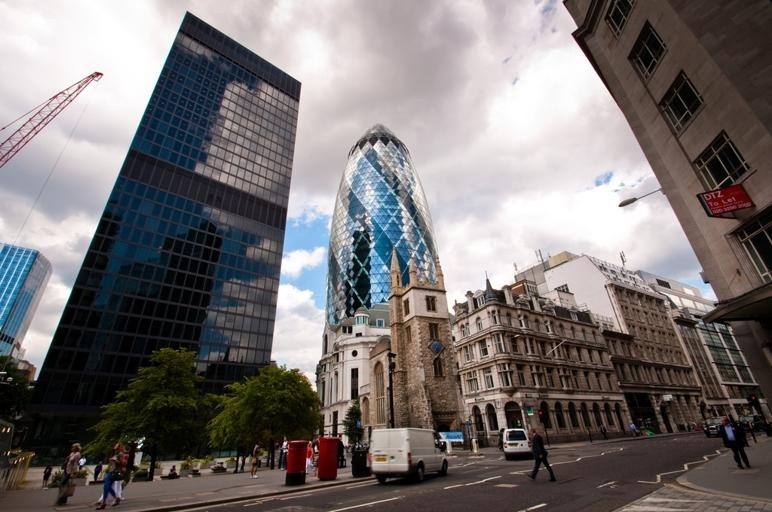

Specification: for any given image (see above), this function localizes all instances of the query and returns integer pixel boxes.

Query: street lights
[618,187,663,208]
[387,352,396,427]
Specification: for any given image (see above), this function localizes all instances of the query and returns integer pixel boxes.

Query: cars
[743,414,763,433]
[701,415,730,437]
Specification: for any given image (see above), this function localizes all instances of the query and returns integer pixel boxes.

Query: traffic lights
[746,393,759,405]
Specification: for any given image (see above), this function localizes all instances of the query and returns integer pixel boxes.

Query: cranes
[0,72,104,169]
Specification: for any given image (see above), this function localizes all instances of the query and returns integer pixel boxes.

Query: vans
[502,427,532,460]
[368,428,449,484]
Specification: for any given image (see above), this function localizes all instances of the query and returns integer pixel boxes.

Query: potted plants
[42,465,110,489]
[131,454,239,482]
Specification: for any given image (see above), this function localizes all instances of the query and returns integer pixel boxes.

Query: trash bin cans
[351,450,371,478]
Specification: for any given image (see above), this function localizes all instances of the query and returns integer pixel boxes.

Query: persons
[278,433,345,475]
[718,414,752,470]
[40,463,52,490]
[77,454,86,470]
[526,426,557,483]
[89,439,138,510]
[50,441,82,505]
[248,441,260,480]
